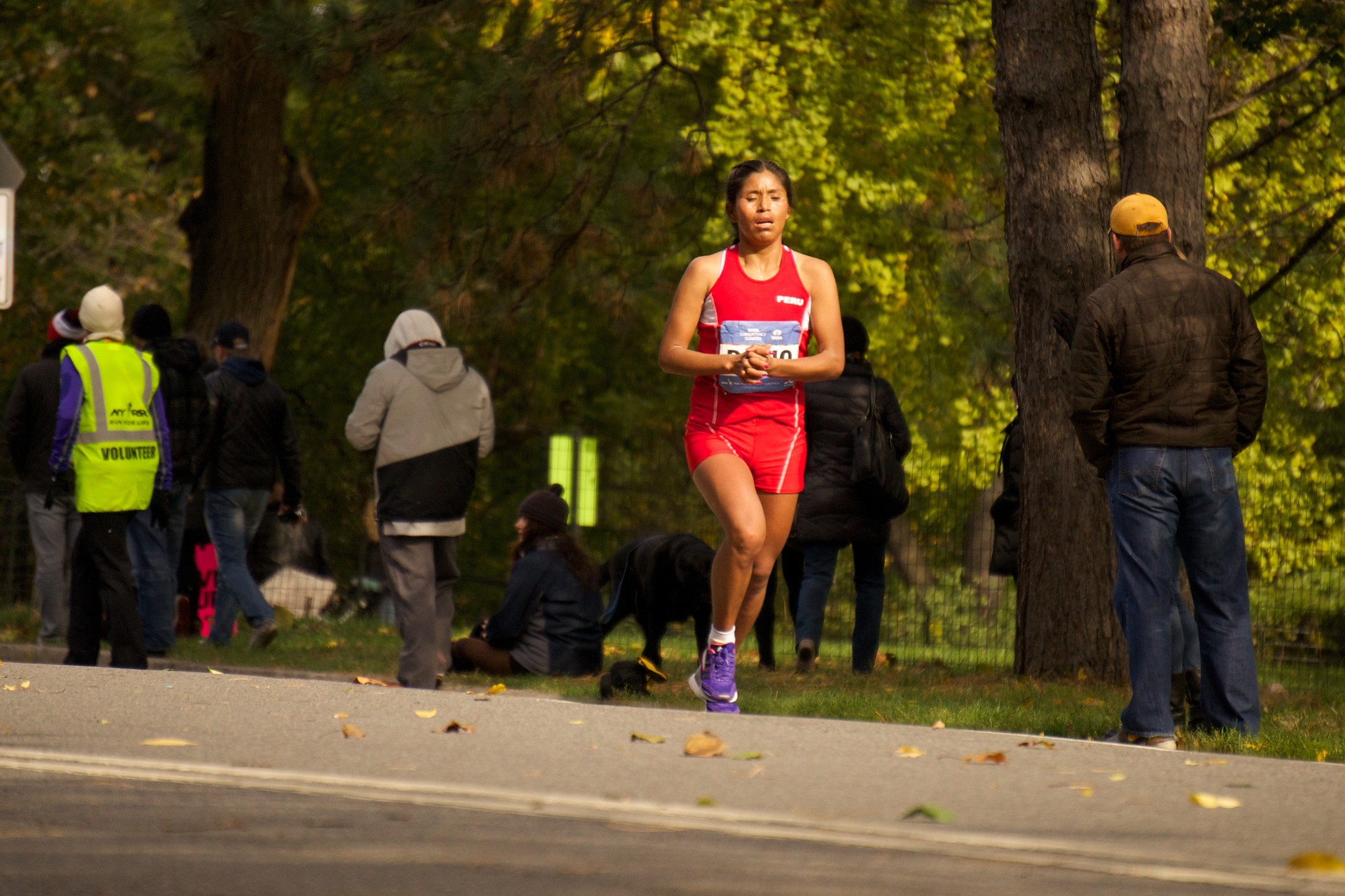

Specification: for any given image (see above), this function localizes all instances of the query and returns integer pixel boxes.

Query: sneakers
[688,665,741,714]
[250,622,279,650]
[796,640,815,673]
[701,642,738,702]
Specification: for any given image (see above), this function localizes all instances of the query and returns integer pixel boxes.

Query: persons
[754,540,800,671]
[1069,190,1267,751]
[795,314,911,675]
[337,494,396,635]
[449,483,603,676]
[596,533,715,680]
[6,284,324,668]
[344,309,496,690]
[658,161,846,714]
[989,374,1020,577]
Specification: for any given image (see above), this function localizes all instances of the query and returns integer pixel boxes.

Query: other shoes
[1185,667,1204,730]
[1170,670,1186,726]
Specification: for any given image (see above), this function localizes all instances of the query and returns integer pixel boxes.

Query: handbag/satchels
[854,375,897,490]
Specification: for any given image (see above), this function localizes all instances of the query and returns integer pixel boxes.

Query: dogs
[601,530,718,670]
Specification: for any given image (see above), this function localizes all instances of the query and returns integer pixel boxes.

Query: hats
[48,308,87,344]
[131,304,170,340]
[521,483,569,532]
[209,323,250,350]
[78,285,125,333]
[383,310,446,359]
[1108,191,1169,237]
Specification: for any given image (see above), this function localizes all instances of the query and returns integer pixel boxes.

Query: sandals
[1106,728,1178,750]
